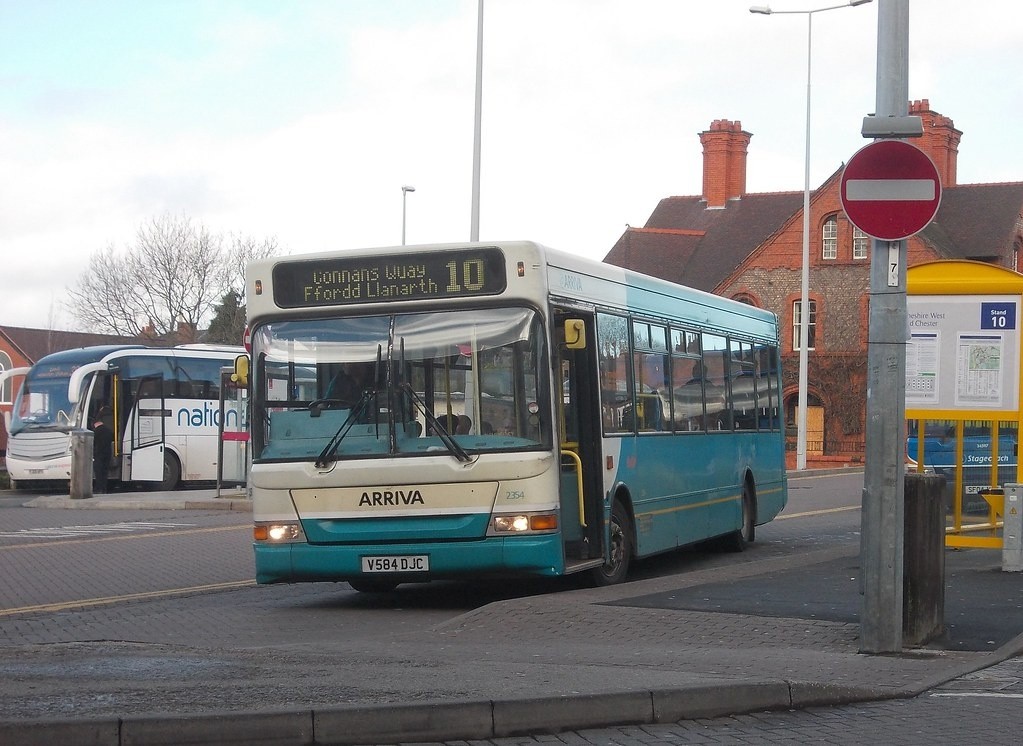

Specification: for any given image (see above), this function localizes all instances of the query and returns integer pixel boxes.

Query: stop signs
[243,324,272,356]
[839,140,943,241]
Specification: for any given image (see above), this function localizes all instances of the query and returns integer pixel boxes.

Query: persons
[429,414,472,436]
[94,419,113,494]
[317,345,369,423]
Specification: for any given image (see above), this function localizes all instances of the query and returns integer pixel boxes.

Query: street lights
[400,185,416,245]
[749,0,875,470]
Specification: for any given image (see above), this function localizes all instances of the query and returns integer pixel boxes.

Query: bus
[231,238,789,597]
[906,419,1017,514]
[0,342,317,494]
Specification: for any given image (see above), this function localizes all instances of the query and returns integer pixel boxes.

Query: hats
[435,415,459,435]
[91,417,104,428]
